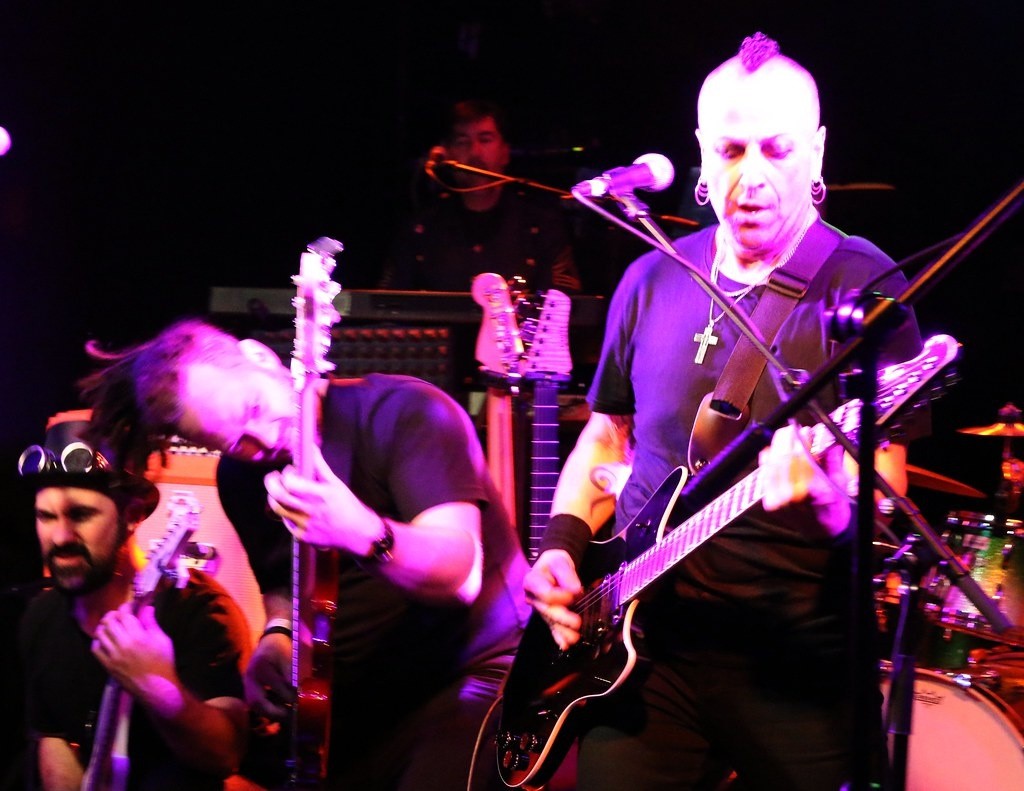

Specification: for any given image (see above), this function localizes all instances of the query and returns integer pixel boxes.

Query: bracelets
[536,513,593,568]
[260,619,292,640]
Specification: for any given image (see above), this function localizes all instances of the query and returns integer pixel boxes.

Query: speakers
[132,448,266,648]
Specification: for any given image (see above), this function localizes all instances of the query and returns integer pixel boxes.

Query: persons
[134,319,532,791]
[397,105,581,311]
[0,421,253,791]
[522,33,925,791]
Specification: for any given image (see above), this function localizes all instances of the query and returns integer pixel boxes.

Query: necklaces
[693,211,811,364]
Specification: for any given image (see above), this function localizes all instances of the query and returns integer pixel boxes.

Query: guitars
[492,330,974,790]
[83,491,201,790]
[288,233,349,791]
[397,270,582,790]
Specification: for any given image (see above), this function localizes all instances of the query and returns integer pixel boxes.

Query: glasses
[16,441,95,475]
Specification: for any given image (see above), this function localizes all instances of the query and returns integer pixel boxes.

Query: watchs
[370,520,393,564]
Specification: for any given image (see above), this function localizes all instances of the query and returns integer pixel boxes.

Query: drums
[920,506,1023,651]
[730,657,1024,791]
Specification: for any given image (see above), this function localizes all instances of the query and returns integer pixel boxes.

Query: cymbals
[955,420,1024,437]
[906,462,987,500]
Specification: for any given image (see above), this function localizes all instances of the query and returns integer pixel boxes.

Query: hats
[15,407,160,525]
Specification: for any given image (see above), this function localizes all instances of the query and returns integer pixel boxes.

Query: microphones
[571,153,675,196]
[425,146,447,169]
[182,542,217,561]
